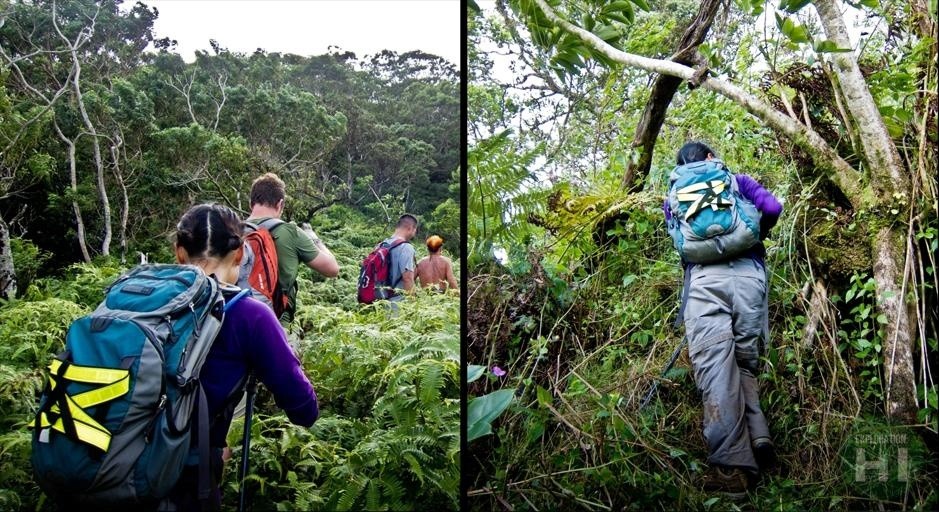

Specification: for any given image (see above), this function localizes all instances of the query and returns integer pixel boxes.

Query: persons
[229,172,340,422]
[413,234,458,293]
[39,200,321,510]
[373,214,419,302]
[663,141,784,499]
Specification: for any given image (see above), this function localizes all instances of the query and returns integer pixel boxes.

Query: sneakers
[692,463,750,501]
[754,437,783,471]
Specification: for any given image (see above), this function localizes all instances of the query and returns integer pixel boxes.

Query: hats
[427,234,443,251]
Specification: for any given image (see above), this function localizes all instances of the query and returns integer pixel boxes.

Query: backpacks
[665,158,763,264]
[29,261,249,507]
[356,237,412,305]
[237,216,292,322]
